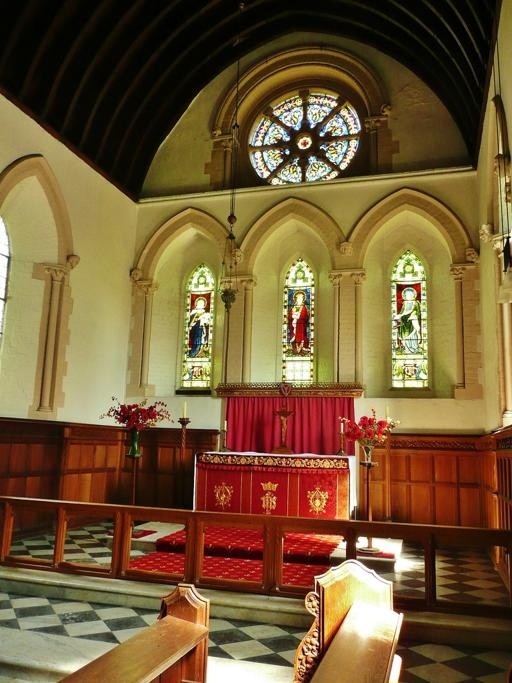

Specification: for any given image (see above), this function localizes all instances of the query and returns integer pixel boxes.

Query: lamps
[214,2,240,313]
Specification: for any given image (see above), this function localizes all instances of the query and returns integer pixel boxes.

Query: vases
[129,428,140,454]
[358,438,378,462]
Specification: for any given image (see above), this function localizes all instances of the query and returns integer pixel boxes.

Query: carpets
[128,523,345,589]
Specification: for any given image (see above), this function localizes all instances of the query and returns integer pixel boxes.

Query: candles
[340,423,343,433]
[223,420,227,430]
[183,401,187,418]
[385,400,390,416]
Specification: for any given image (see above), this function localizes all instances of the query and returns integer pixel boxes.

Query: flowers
[337,408,401,444]
[96,394,176,434]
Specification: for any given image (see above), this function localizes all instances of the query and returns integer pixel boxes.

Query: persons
[189,297,210,357]
[397,288,421,352]
[288,292,309,354]
